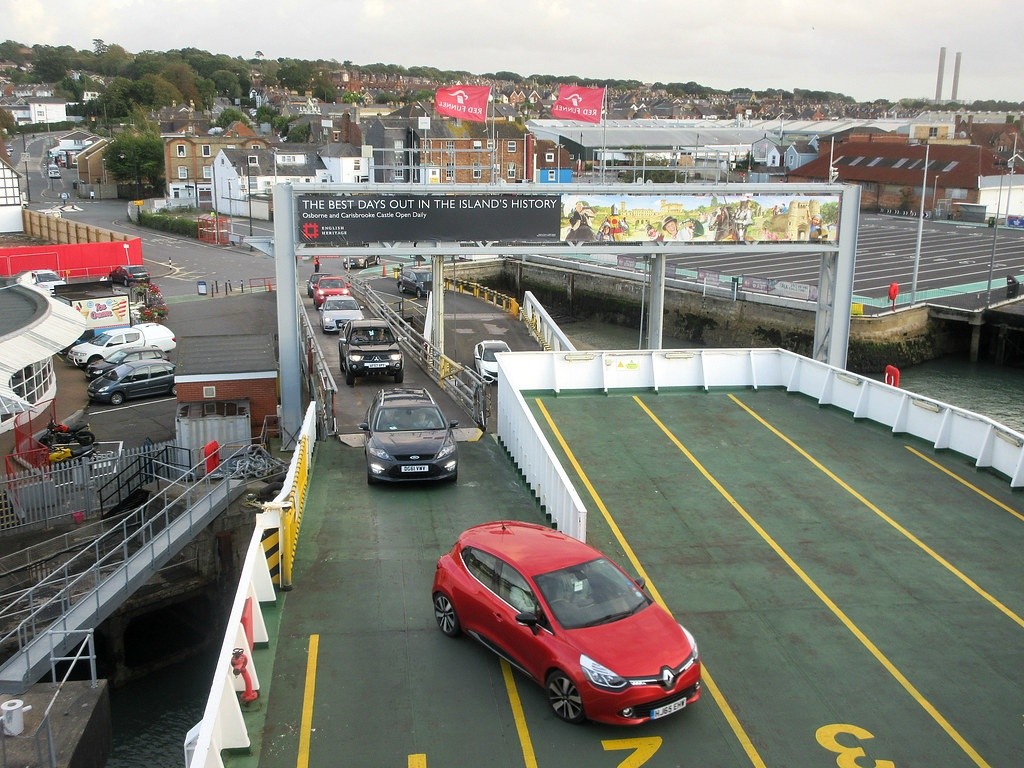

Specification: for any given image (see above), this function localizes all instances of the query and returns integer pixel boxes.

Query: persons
[509,563,596,616]
[381,410,435,430]
[561,199,829,242]
[314,258,320,273]
[352,328,388,341]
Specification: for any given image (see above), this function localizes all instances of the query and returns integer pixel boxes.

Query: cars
[305,273,335,298]
[313,277,352,310]
[32,272,66,296]
[319,296,364,334]
[72,179,85,189]
[343,255,380,269]
[473,340,512,383]
[47,165,61,178]
[109,265,151,287]
[87,359,177,405]
[432,520,701,725]
[338,318,405,385]
[396,270,447,299]
[85,347,170,380]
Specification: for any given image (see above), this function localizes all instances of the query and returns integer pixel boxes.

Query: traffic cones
[381,265,387,277]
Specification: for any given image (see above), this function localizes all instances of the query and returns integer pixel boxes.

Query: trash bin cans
[1006,275,1019,298]
[988,217,995,227]
[197,281,207,295]
[732,277,738,291]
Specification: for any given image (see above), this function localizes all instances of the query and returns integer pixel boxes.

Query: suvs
[360,388,459,485]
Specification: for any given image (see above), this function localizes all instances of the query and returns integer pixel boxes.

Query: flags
[551,84,605,125]
[436,85,492,122]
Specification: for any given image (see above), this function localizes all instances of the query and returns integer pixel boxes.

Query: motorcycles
[39,414,100,470]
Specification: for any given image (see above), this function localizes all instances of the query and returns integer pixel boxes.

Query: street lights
[1004,133,1018,227]
[17,131,31,201]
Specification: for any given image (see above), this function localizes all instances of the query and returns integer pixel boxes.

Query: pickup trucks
[66,323,176,369]
